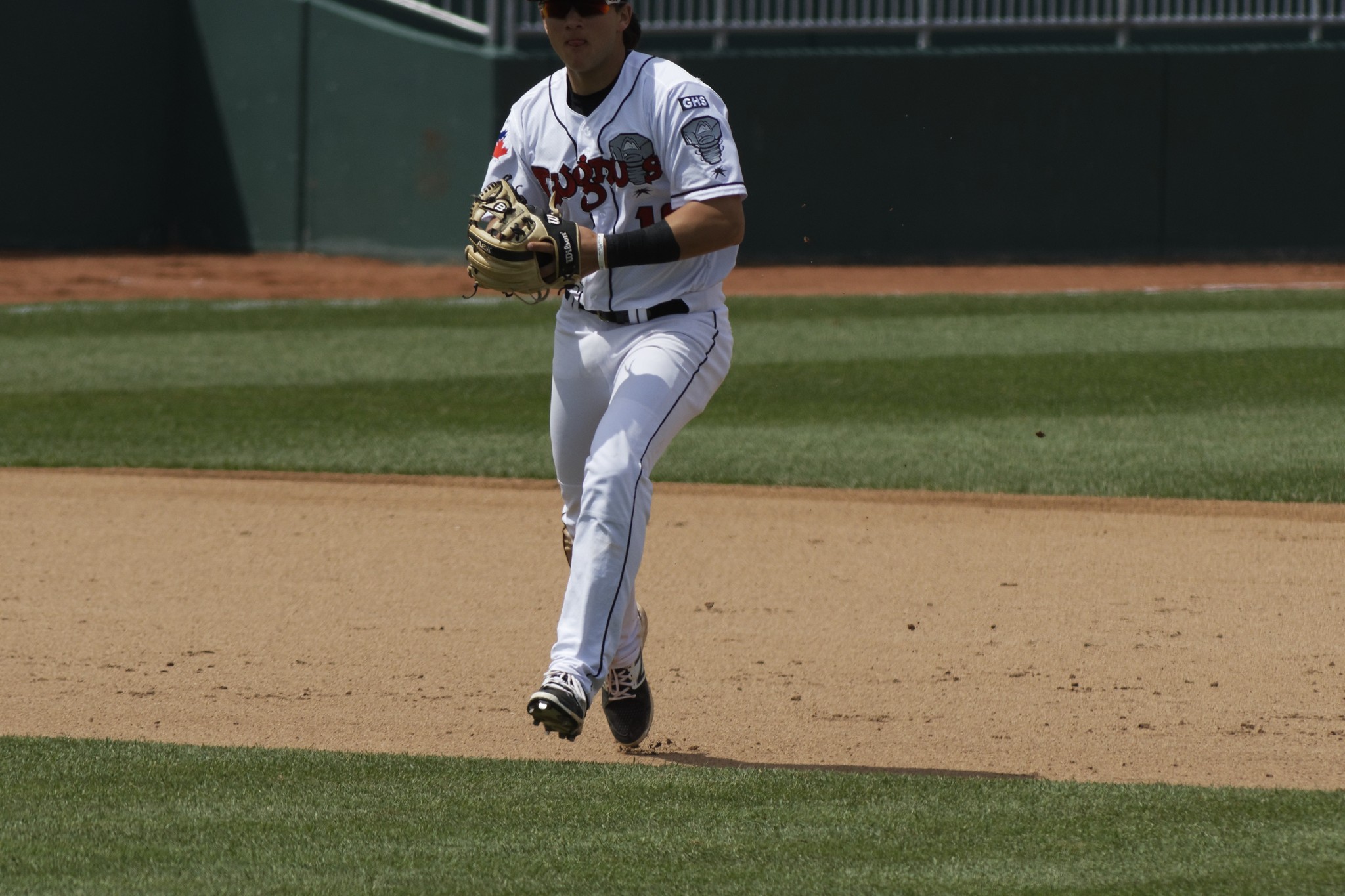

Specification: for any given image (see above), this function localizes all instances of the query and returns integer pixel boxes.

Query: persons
[462,0,749,746]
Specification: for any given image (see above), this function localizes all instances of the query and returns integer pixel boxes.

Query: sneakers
[601,602,654,746]
[527,670,589,742]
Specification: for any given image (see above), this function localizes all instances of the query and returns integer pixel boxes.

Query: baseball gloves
[465,180,586,298]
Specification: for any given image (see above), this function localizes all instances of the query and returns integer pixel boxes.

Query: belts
[565,288,689,323]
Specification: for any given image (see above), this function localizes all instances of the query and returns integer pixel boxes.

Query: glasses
[539,0,621,17]
